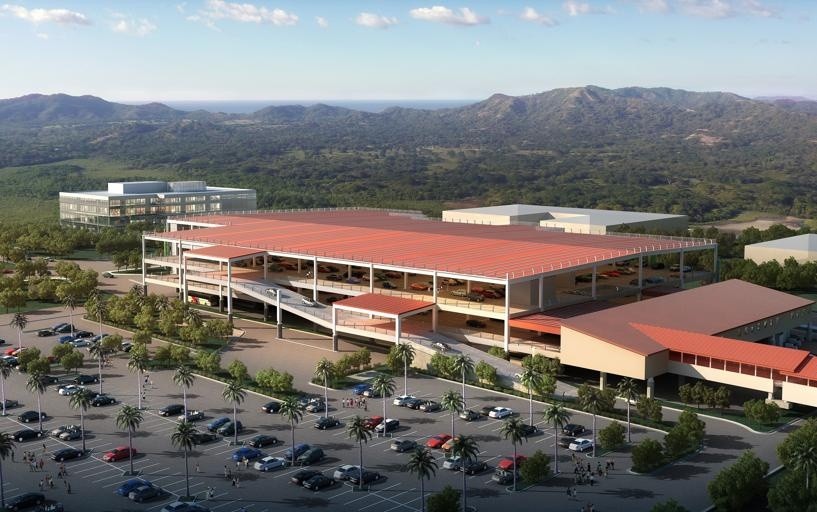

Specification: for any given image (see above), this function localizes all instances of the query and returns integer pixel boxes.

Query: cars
[160,501,210,512]
[231,446,262,462]
[40,375,58,386]
[301,297,318,307]
[2,346,29,369]
[348,470,380,485]
[248,434,277,448]
[53,383,107,402]
[52,424,81,441]
[298,398,327,413]
[364,396,593,484]
[313,416,340,430]
[18,411,47,423]
[264,287,278,296]
[102,445,138,463]
[289,468,335,492]
[431,340,452,352]
[382,281,397,289]
[51,447,82,463]
[353,384,390,397]
[36,321,134,354]
[401,272,417,277]
[411,277,505,303]
[206,416,231,432]
[574,260,690,290]
[117,477,163,502]
[284,443,311,461]
[253,456,286,473]
[326,273,343,281]
[466,320,486,328]
[5,492,64,512]
[234,257,403,290]
[784,322,817,349]
[11,428,42,443]
[46,355,61,363]
[177,410,205,423]
[296,447,324,466]
[158,403,186,417]
[0,339,6,343]
[0,399,19,410]
[261,402,281,414]
[89,395,116,407]
[73,373,98,385]
[216,419,242,436]
[333,464,364,480]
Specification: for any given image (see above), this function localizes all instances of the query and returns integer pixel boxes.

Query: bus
[188,291,219,307]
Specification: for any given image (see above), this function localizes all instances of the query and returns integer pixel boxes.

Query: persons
[99,355,154,401]
[565,452,615,512]
[22,443,72,496]
[340,394,369,410]
[191,454,251,500]
